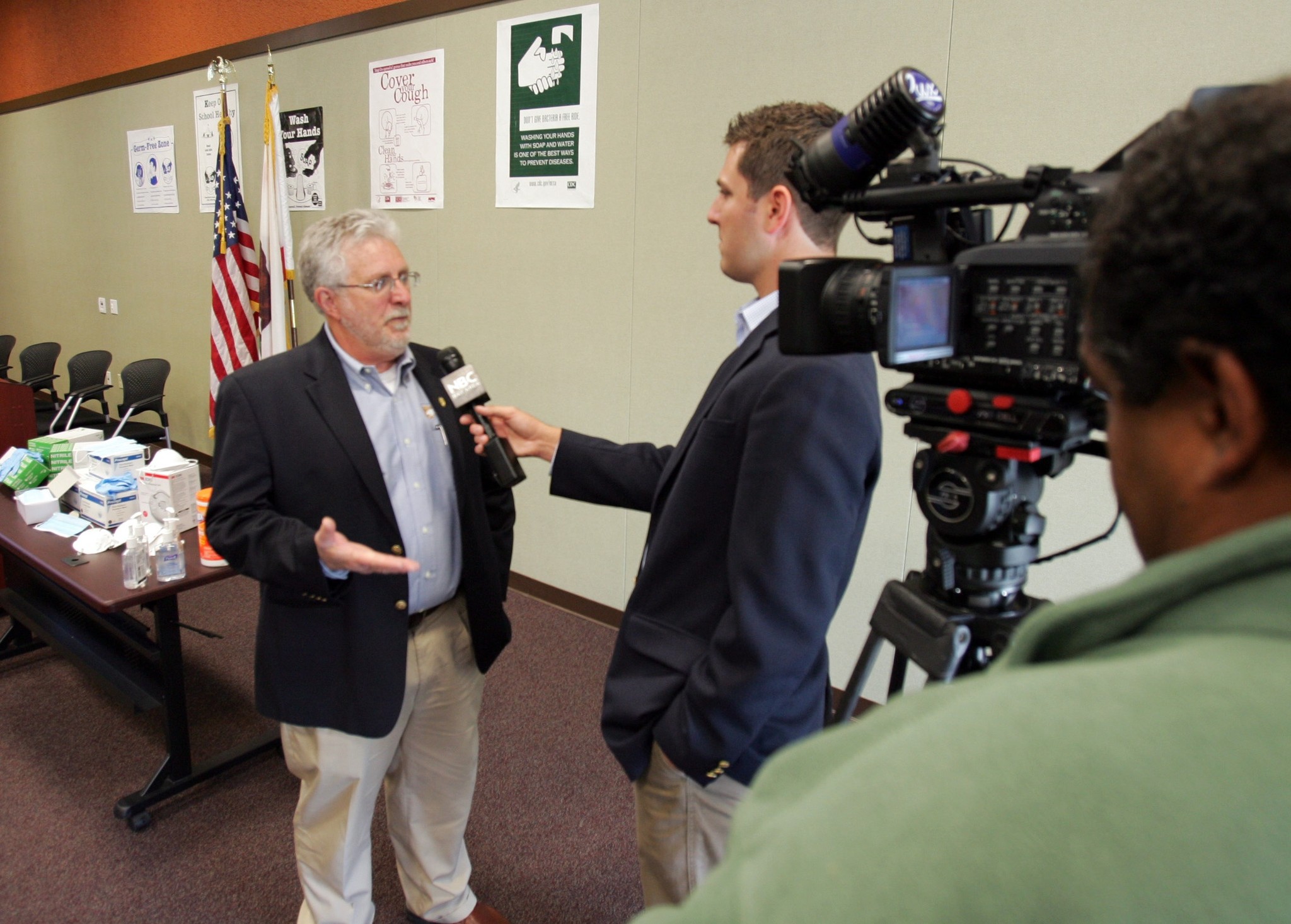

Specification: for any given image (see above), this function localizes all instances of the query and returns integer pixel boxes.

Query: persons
[460,101,881,908]
[205,207,516,924]
[628,77,1291,924]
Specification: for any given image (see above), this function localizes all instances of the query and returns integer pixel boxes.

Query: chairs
[15,341,62,414]
[86,358,171,449]
[36,350,114,438]
[0,334,16,379]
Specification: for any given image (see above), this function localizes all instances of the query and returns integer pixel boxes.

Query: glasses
[339,267,419,296]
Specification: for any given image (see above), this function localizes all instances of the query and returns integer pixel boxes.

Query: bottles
[196,487,231,568]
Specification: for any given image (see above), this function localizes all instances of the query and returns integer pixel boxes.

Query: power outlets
[105,371,111,385]
[117,374,124,389]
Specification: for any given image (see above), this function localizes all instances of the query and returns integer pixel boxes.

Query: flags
[209,80,299,435]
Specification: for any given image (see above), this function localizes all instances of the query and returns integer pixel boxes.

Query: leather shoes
[406,888,509,924]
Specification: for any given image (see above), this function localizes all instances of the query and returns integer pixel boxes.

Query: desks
[0,443,282,836]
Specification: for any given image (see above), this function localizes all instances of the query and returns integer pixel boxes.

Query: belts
[406,602,447,629]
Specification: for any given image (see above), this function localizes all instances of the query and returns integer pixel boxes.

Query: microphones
[437,346,526,488]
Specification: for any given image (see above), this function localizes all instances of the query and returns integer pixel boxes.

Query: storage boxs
[135,462,202,531]
[27,426,103,468]
[0,446,51,491]
[91,442,145,480]
[49,442,107,511]
[14,464,81,526]
[79,479,139,529]
